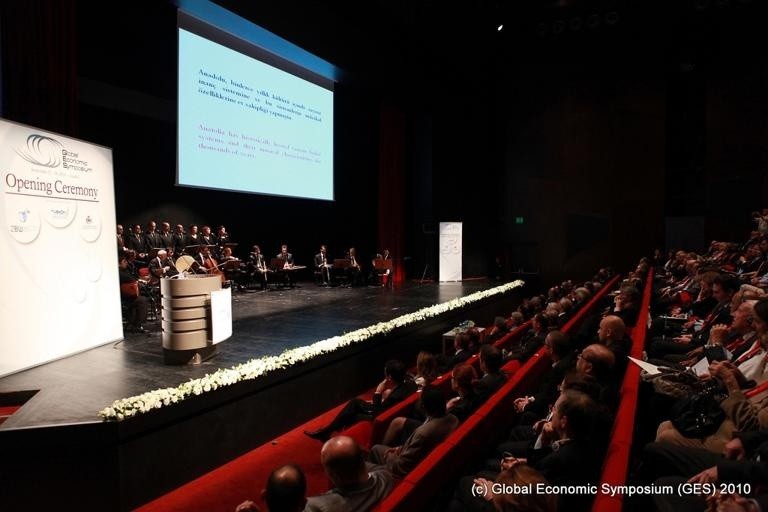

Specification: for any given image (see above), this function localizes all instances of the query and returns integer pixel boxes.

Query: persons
[408,349,441,392]
[339,247,360,288]
[233,461,348,511]
[366,383,458,481]
[248,243,274,291]
[318,434,395,512]
[276,244,294,286]
[302,358,418,444]
[116,218,246,336]
[370,248,392,288]
[444,202,766,511]
[312,244,333,286]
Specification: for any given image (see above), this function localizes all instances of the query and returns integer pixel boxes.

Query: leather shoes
[304,431,331,443]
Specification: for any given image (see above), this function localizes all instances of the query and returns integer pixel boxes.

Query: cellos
[204,250,226,284]
